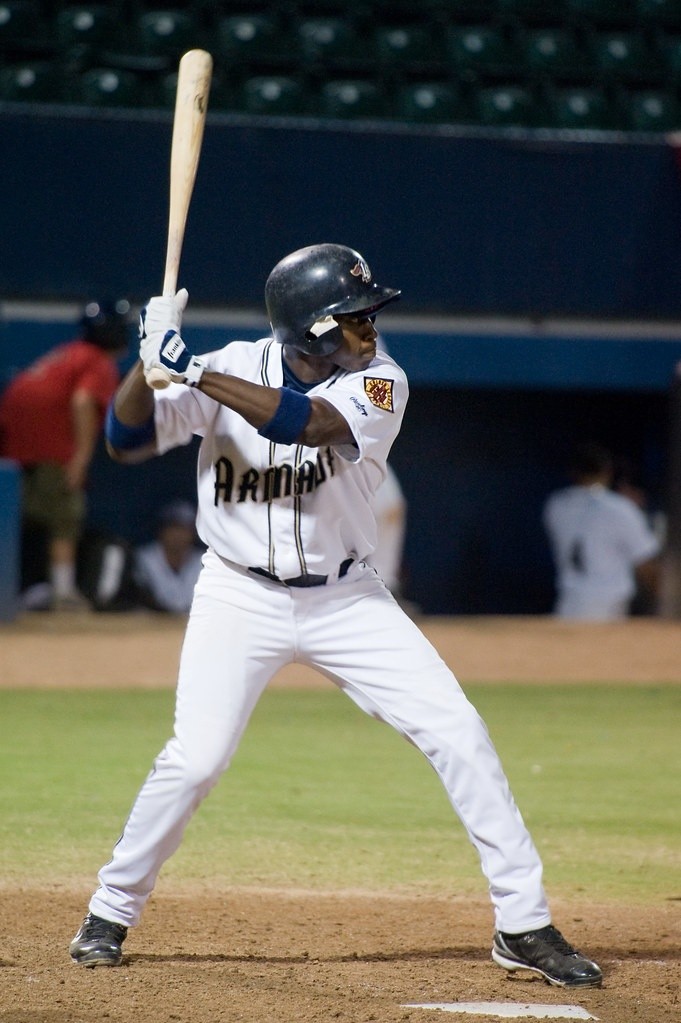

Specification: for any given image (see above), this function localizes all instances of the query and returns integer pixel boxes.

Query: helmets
[264,243,401,357]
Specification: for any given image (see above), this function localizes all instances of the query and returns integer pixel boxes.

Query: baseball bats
[145,48,215,390]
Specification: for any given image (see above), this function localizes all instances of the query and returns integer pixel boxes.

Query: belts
[248,558,355,588]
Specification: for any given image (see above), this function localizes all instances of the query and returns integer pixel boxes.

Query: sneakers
[492,924,602,988]
[69,912,128,967]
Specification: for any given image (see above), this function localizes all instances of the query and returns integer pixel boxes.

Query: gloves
[138,288,188,339]
[139,329,204,388]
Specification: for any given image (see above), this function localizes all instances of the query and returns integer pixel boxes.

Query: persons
[540,437,681,618]
[72,244,604,990]
[0,302,207,614]
